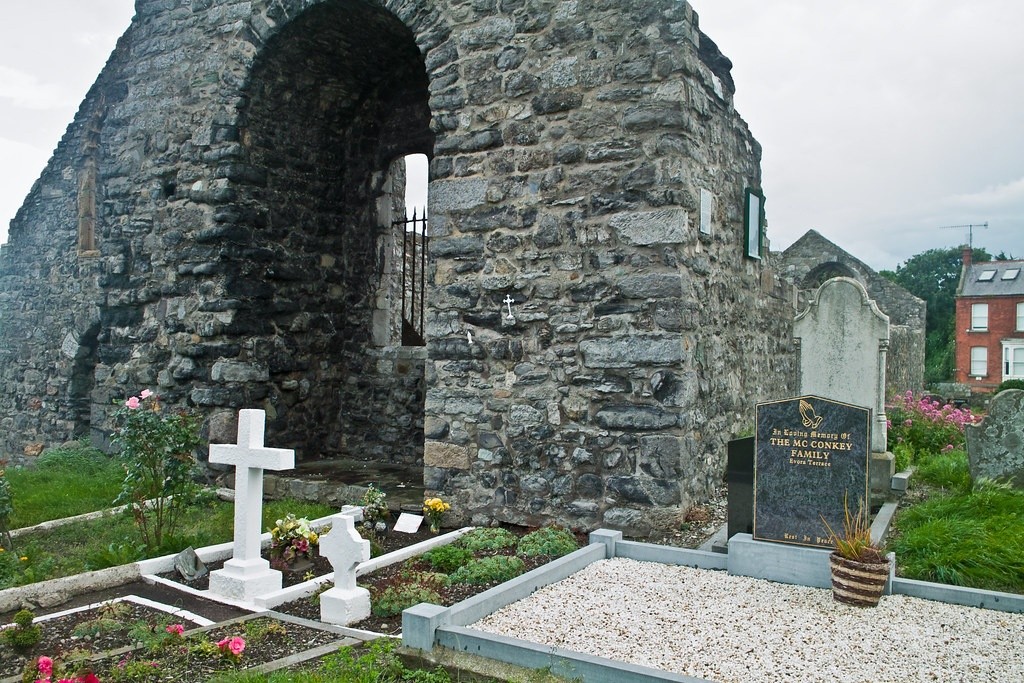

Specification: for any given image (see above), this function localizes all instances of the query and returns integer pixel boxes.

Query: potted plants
[819,486,893,608]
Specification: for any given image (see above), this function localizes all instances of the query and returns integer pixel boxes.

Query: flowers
[424,499,452,529]
[267,512,320,568]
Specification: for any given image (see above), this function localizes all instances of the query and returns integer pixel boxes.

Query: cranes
[942,223,989,256]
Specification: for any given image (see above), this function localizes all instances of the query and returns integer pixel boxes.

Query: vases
[280,556,314,573]
[428,517,441,534]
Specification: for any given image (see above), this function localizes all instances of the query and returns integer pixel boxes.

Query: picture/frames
[743,187,764,261]
